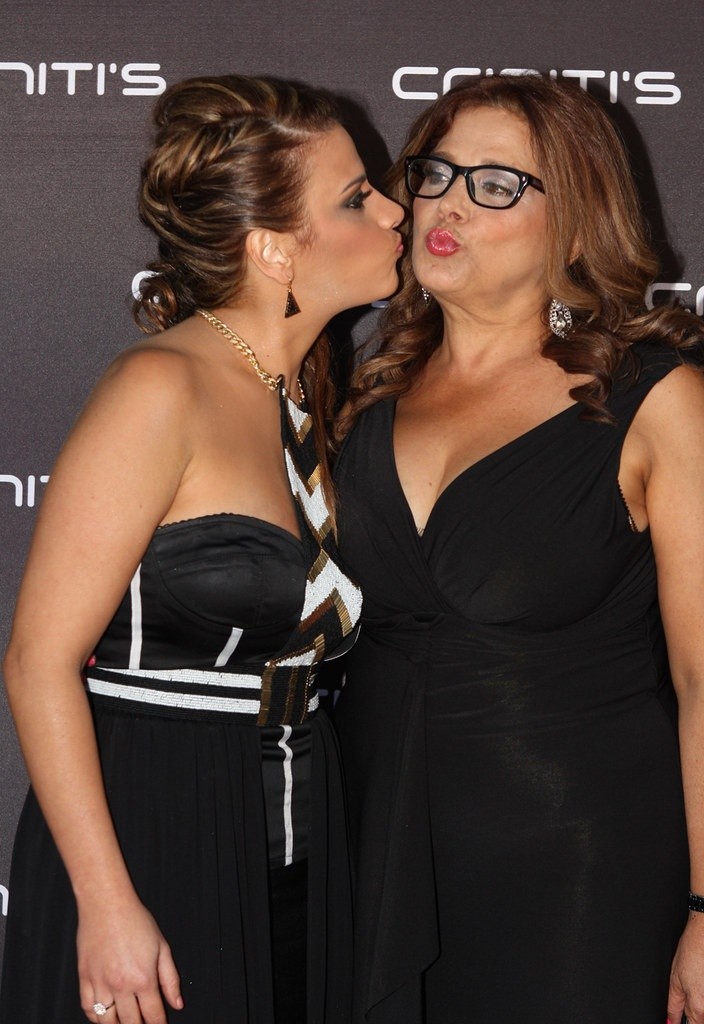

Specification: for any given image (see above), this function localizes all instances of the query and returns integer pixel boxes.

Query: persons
[0,72,406,1024]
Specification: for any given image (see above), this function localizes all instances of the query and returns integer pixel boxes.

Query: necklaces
[192,307,306,408]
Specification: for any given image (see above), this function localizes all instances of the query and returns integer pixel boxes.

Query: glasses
[405,149,547,211]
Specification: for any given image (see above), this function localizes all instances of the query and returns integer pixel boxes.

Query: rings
[93,1002,115,1016]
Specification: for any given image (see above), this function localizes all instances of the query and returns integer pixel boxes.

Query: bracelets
[328,72,704,1024]
[688,890,704,912]
